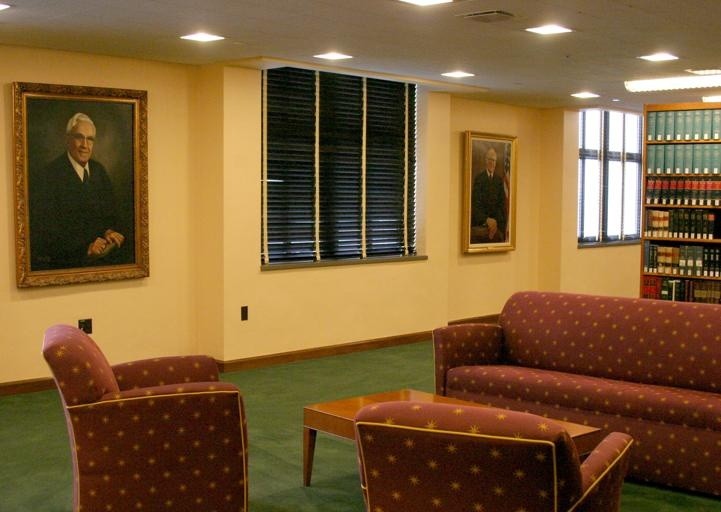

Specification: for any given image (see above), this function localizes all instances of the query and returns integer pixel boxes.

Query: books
[642,111,721,304]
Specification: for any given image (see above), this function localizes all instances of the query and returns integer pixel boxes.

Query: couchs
[429,291,720,499]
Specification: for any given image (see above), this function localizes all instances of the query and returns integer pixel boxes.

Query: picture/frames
[462,129,519,256]
[9,79,156,289]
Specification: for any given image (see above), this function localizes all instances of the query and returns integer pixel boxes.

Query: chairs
[41,320,250,512]
[349,401,635,510]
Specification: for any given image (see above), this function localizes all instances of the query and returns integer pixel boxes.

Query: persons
[37,111,125,271]
[472,147,506,243]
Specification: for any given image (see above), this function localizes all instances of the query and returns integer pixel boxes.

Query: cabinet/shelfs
[640,99,720,305]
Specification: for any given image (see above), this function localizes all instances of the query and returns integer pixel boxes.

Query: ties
[83,168,88,183]
